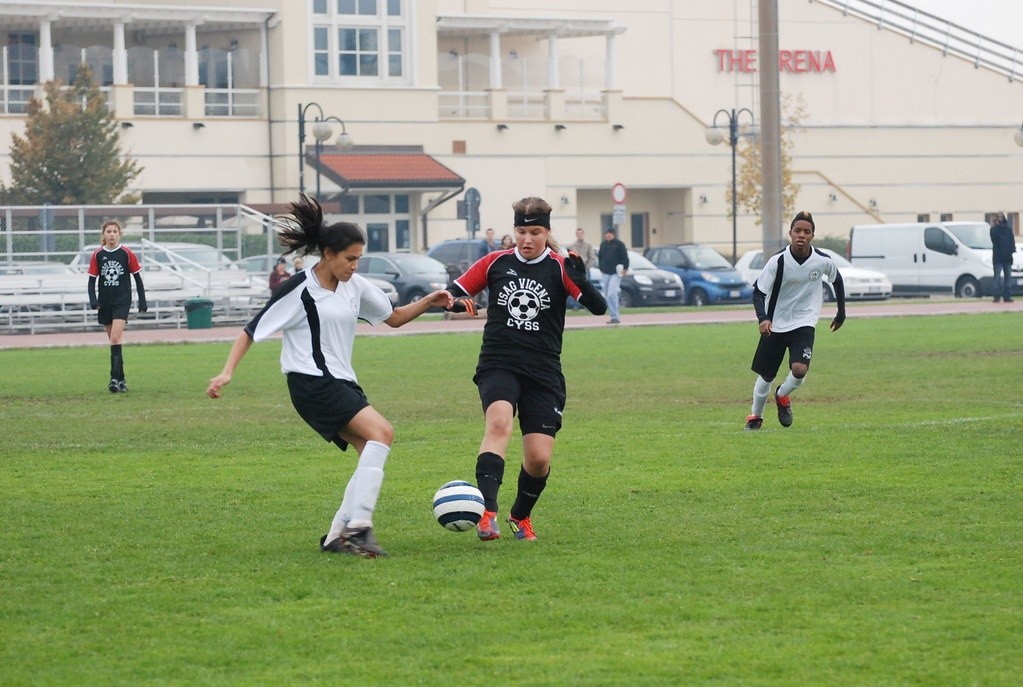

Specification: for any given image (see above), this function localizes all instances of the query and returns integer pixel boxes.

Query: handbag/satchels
[616,263,635,277]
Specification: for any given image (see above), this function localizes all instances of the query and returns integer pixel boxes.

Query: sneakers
[744,415,764,433]
[507,513,537,541]
[338,520,389,558]
[476,509,501,542]
[118,380,128,392]
[774,385,793,427]
[107,379,118,392]
[320,533,343,552]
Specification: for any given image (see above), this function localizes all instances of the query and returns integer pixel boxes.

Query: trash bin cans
[184,295,215,328]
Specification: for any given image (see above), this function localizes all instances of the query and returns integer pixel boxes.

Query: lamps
[497,125,507,128]
[613,124,623,128]
[555,125,565,129]
[193,122,205,128]
[121,122,133,128]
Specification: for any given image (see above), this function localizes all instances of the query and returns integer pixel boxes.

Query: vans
[848,220,1023,298]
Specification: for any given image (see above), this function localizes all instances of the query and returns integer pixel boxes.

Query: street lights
[298,102,352,204]
[706,108,758,264]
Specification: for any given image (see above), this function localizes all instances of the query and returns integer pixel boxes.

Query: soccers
[432,480,485,532]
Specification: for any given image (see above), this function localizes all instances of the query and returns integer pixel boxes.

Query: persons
[478,228,498,259]
[498,235,515,250]
[441,197,608,543]
[598,227,629,324]
[269,257,290,290]
[570,227,595,279]
[204,193,453,556]
[990,213,1016,303]
[288,256,304,275]
[744,211,846,430]
[86,220,148,393]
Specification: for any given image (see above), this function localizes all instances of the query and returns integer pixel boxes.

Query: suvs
[596,249,686,307]
[734,246,892,303]
[425,240,503,309]
[156,242,273,309]
[70,244,183,306]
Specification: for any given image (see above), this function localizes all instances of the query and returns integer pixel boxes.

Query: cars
[1009,242,1023,287]
[359,253,449,309]
[558,246,607,310]
[0,261,93,311]
[235,254,401,311]
[642,245,754,306]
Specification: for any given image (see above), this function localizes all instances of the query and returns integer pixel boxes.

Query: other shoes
[1004,298,1013,302]
[992,299,999,302]
[607,317,620,323]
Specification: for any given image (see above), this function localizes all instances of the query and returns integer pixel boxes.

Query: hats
[294,258,303,265]
[604,226,615,235]
[789,211,816,235]
[277,257,285,265]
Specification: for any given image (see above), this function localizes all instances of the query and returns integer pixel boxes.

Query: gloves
[138,303,147,313]
[90,301,99,309]
[449,295,482,318]
[563,246,591,289]
